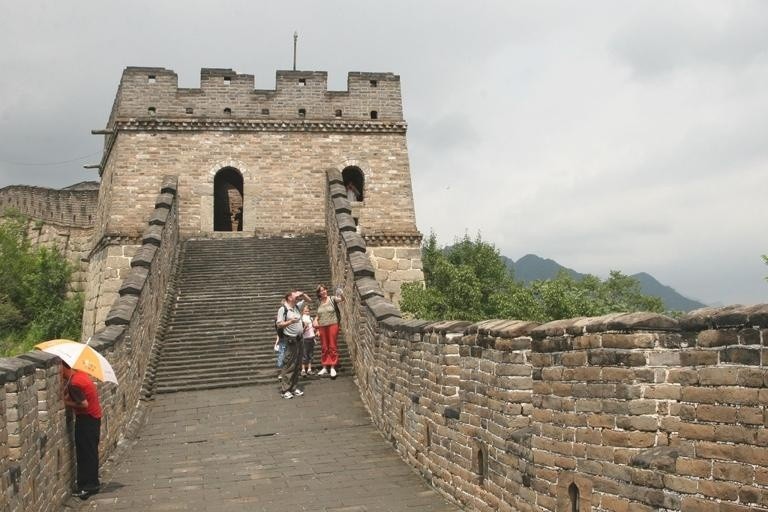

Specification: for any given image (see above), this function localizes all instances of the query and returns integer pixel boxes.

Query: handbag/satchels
[275,305,287,339]
[333,303,340,321]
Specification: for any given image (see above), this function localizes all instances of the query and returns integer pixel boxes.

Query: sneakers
[300,370,315,376]
[318,368,336,376]
[281,389,304,398]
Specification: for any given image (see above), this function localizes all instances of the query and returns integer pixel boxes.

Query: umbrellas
[34,334,120,401]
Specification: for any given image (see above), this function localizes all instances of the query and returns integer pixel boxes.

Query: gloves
[336,288,343,295]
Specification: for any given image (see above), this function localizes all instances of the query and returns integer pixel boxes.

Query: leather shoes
[72,484,100,497]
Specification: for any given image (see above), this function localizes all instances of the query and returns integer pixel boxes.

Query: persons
[272,283,346,399]
[346,181,360,202]
[61,366,105,497]
